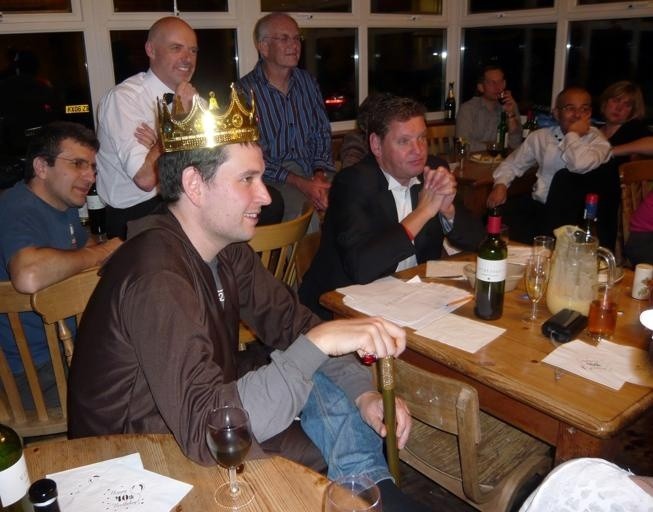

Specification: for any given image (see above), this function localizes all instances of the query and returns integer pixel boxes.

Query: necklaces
[638,474,652,487]
[604,123,618,139]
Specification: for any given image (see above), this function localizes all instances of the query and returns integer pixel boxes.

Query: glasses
[54,156,98,173]
[262,33,304,44]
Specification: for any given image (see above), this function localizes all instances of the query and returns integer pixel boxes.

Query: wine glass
[520,236,555,326]
[488,144,502,169]
[497,92,514,117]
[206,406,256,510]
[454,137,472,173]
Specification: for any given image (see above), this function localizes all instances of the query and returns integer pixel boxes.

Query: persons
[298,97,487,319]
[92,18,285,266]
[515,407,653,511]
[597,80,653,159]
[67,82,431,511]
[486,86,613,241]
[625,191,652,269]
[0,120,125,408]
[339,92,390,172]
[454,66,522,152]
[232,12,334,255]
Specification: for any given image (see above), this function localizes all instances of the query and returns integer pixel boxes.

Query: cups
[88,227,109,245]
[586,282,619,339]
[632,264,653,301]
[324,474,383,512]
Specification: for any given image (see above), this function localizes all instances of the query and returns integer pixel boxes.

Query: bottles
[475,208,507,320]
[497,111,509,155]
[579,194,604,247]
[444,81,456,122]
[0,423,61,512]
[522,110,538,143]
[86,183,108,227]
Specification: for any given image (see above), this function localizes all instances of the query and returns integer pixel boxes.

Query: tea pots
[546,225,616,317]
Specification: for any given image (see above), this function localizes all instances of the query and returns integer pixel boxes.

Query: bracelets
[506,114,516,119]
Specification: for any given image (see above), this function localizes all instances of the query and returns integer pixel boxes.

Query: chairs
[0,125,653,512]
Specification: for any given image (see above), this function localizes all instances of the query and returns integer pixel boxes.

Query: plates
[467,151,504,163]
[640,309,653,331]
[463,247,625,292]
